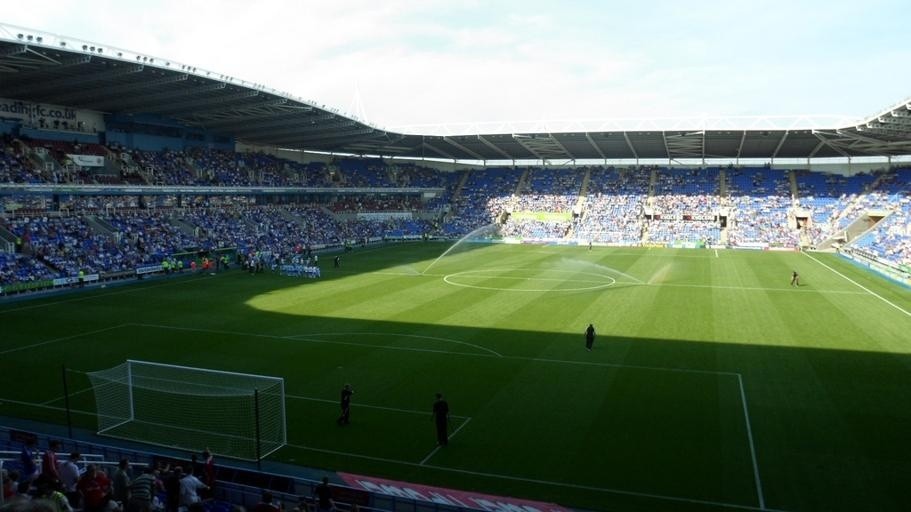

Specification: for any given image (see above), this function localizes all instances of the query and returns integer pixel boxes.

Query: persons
[584,323,596,352]
[1,136,449,287]
[1,440,217,511]
[257,475,337,511]
[337,382,356,427]
[427,162,911,287]
[431,391,450,450]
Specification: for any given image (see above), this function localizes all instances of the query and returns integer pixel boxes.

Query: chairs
[0,134,444,289]
[442,167,911,274]
